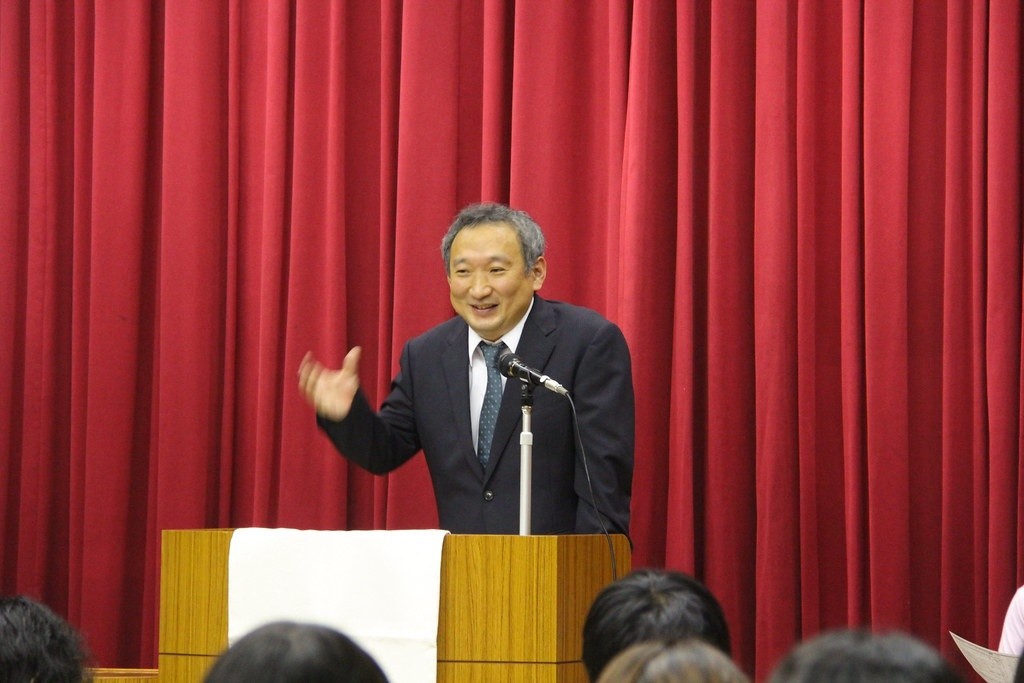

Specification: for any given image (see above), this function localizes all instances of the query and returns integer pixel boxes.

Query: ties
[477,341,509,474]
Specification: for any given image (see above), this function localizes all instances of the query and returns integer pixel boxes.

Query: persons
[1,570,1024,683]
[298,201,634,537]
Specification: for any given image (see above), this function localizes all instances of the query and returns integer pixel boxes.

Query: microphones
[498,353,568,395]
